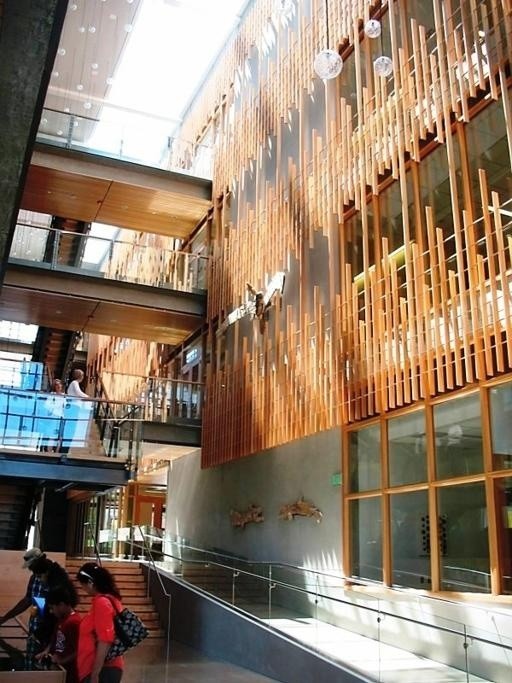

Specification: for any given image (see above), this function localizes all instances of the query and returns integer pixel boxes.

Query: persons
[0,545,78,670]
[58,368,91,453]
[38,377,67,452]
[32,584,86,682]
[74,562,126,682]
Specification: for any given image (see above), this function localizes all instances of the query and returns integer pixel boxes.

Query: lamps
[314,0,343,80]
[56,455,67,464]
[373,0,393,77]
[364,0,381,38]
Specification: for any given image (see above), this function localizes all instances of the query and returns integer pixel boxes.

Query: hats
[21,548,46,569]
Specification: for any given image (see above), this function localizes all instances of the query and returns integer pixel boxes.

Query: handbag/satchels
[94,595,150,661]
